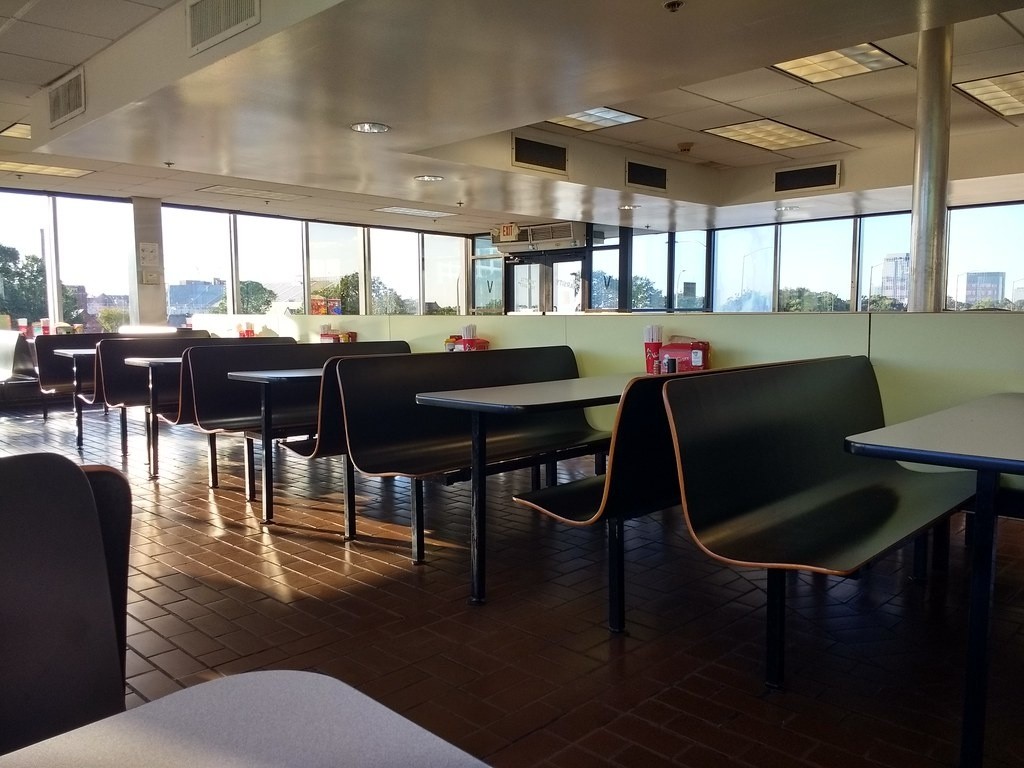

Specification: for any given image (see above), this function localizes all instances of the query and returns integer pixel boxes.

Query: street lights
[740,247,767,295]
[868,263,883,312]
[954,272,968,311]
[1011,279,1024,312]
[677,270,688,306]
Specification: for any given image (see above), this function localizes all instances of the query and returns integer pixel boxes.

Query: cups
[41,326,50,335]
[245,330,254,337]
[18,326,28,339]
[645,342,662,373]
[463,339,477,352]
[339,335,348,342]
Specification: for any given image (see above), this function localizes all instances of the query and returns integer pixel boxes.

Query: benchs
[99,337,297,457]
[189,341,411,503]
[34,333,120,419]
[13,332,48,420]
[278,355,541,542]
[74,342,106,448]
[0,453,134,760]
[0,329,19,384]
[513,356,853,633]
[148,348,192,480]
[337,346,615,565]
[664,356,979,689]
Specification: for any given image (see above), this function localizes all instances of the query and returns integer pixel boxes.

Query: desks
[124,356,183,465]
[54,348,99,421]
[416,373,645,605]
[0,671,491,768]
[227,367,324,525]
[844,393,1024,768]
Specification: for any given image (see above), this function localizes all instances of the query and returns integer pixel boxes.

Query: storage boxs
[18,326,34,339]
[659,340,710,374]
[239,330,254,337]
[320,334,340,343]
[453,338,489,352]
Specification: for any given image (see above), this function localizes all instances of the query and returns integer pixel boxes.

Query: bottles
[653,361,661,374]
